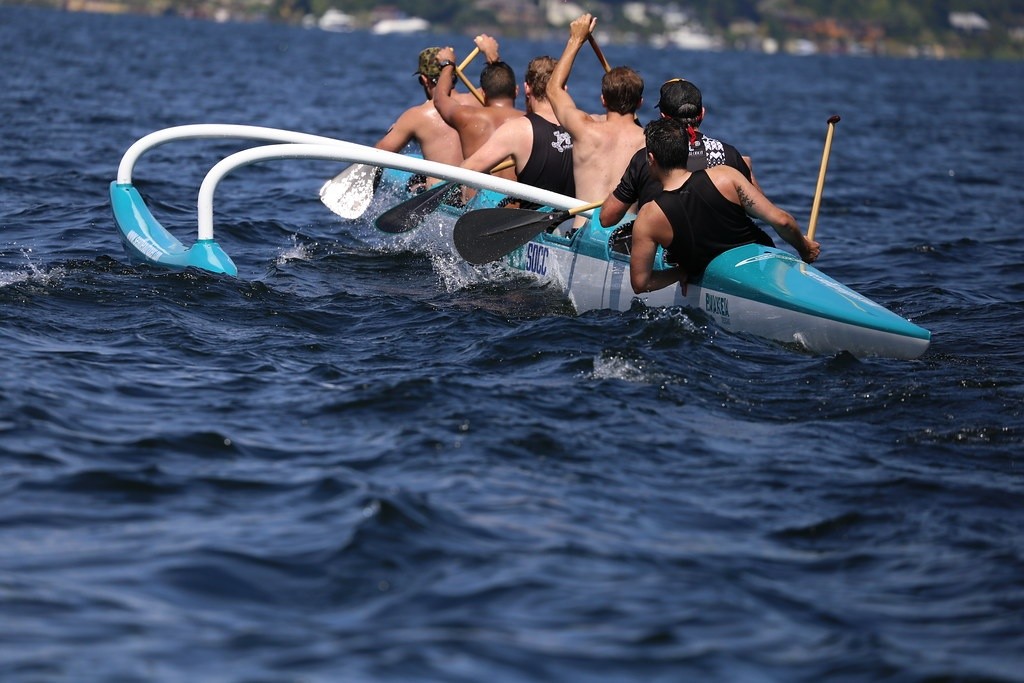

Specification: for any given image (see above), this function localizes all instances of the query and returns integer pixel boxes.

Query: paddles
[315,45,482,221]
[450,197,619,266]
[453,65,484,104]
[458,65,490,104]
[806,114,840,246]
[374,158,519,236]
[582,30,610,74]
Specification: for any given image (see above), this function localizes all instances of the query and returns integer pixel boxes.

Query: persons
[630,117,821,295]
[434,45,530,209]
[371,33,502,207]
[544,13,649,240]
[598,78,766,228]
[459,53,609,212]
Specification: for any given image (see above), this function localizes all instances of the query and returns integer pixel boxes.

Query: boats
[110,122,936,360]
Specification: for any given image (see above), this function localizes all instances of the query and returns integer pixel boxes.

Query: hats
[412,47,456,76]
[654,78,703,119]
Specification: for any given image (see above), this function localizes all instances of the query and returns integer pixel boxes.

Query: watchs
[438,59,455,71]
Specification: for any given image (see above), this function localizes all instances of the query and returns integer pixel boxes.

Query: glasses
[418,76,424,86]
